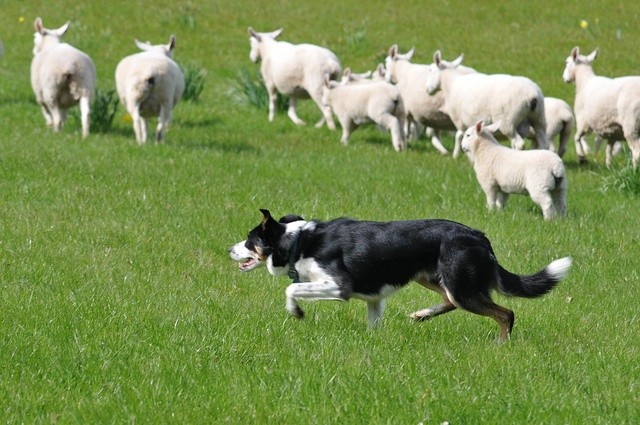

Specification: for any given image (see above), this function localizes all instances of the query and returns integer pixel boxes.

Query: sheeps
[372,62,419,138]
[341,66,372,87]
[320,61,405,151]
[384,44,486,156]
[29,16,97,139]
[425,49,550,159]
[562,44,639,167]
[498,96,573,159]
[460,119,568,219]
[247,26,341,130]
[114,33,185,148]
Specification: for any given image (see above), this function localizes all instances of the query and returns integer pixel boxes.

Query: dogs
[226,207,575,347]
